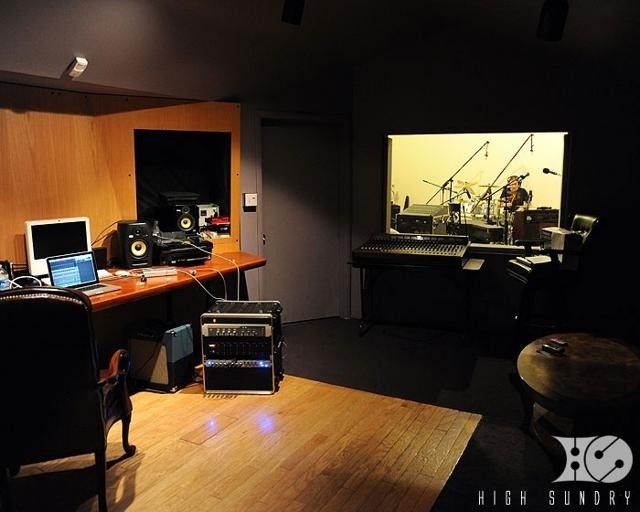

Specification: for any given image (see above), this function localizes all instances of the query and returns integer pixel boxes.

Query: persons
[498,176,528,213]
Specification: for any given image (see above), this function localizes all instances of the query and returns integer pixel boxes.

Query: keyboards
[41,269,114,287]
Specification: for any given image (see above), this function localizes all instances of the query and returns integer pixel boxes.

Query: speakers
[484,141,489,157]
[543,168,558,175]
[159,192,200,238]
[519,172,530,182]
[92,247,107,270]
[117,221,152,268]
[530,134,534,152]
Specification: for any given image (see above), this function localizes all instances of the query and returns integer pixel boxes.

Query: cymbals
[516,205,535,208]
[479,185,498,187]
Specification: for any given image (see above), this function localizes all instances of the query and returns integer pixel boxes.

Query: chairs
[507,214,598,331]
[1,288,135,512]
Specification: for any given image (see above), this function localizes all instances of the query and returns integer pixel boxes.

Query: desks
[87,251,266,312]
[518,333,640,435]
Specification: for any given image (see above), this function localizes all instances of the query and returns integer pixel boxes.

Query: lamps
[62,55,89,81]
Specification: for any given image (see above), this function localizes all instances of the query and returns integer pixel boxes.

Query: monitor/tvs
[24,216,92,276]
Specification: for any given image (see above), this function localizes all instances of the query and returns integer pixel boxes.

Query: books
[142,265,177,277]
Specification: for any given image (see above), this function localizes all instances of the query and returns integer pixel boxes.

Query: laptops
[47,251,121,297]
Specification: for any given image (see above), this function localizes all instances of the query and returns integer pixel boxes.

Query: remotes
[542,343,564,356]
